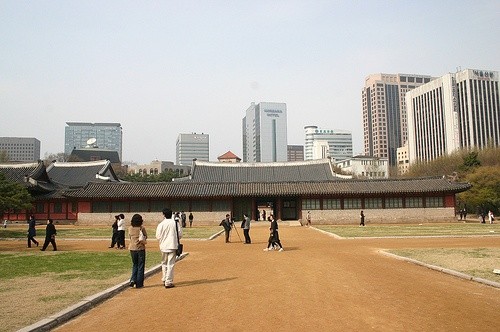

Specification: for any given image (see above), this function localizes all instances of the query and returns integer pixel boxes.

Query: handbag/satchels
[139,226,147,244]
[175,244,183,257]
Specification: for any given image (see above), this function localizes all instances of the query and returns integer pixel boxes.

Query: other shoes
[165,283,175,288]
[109,246,113,248]
[279,248,283,251]
[123,247,125,249]
[226,241,231,243]
[120,245,123,249]
[115,247,118,249]
[274,245,276,250]
[40,249,42,251]
[264,248,269,251]
[129,281,134,287]
[276,245,279,249]
[269,247,274,250]
[37,242,39,247]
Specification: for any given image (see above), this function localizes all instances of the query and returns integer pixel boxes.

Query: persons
[243,213,251,244]
[181,211,186,227]
[172,211,181,222]
[4,220,7,228]
[220,214,233,243]
[156,209,182,289]
[262,210,266,221]
[459,209,463,220]
[359,211,367,226]
[480,209,485,223]
[40,219,57,251]
[307,211,311,225]
[257,210,260,221]
[488,210,495,224]
[463,208,467,219]
[109,214,126,250]
[26,215,39,248]
[264,215,285,252]
[128,214,148,289]
[189,213,193,227]
